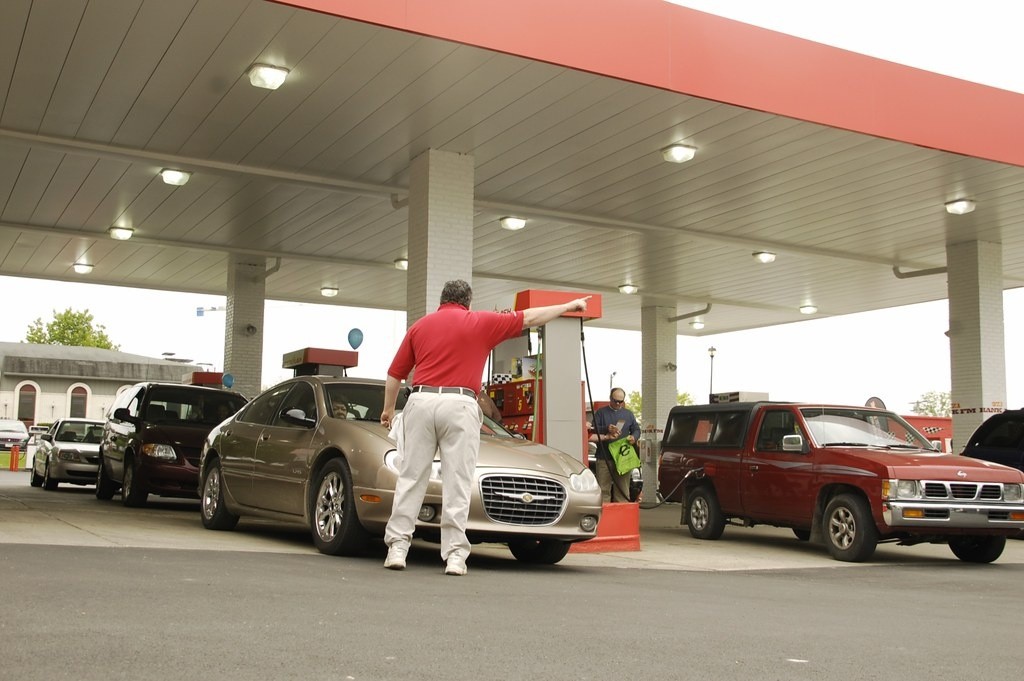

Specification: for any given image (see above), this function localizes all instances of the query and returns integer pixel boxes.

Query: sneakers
[383,547,407,569]
[445,556,468,575]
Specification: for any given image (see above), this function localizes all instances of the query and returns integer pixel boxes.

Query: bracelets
[604,433,606,441]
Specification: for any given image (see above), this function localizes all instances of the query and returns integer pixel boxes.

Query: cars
[0,417,31,451]
[196,374,603,566]
[960,407,1024,541]
[31,417,106,490]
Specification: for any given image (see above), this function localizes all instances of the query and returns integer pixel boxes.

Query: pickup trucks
[656,399,1023,563]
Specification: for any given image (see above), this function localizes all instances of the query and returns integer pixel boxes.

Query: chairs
[149,404,165,418]
[63,431,76,441]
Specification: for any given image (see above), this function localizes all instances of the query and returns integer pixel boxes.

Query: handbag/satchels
[608,437,641,476]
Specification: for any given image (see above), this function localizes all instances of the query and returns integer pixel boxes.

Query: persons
[381,279,592,576]
[331,394,349,419]
[478,390,504,426]
[586,387,641,503]
[207,399,234,423]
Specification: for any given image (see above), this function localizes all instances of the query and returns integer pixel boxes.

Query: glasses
[611,397,624,403]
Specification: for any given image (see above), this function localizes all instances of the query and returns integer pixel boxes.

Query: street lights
[707,345,716,393]
[610,372,617,389]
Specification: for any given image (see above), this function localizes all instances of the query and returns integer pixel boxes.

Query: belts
[412,386,476,399]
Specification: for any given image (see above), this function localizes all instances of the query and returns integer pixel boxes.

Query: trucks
[586,399,625,456]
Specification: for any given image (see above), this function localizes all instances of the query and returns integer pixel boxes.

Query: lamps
[320,287,340,298]
[394,258,409,272]
[73,263,95,274]
[247,64,290,90]
[618,284,639,295]
[108,226,134,240]
[159,167,194,185]
[499,215,527,231]
[659,143,697,163]
[688,321,706,330]
[752,251,777,264]
[944,198,977,215]
[799,305,818,315]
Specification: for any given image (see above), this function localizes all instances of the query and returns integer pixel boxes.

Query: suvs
[95,381,251,509]
